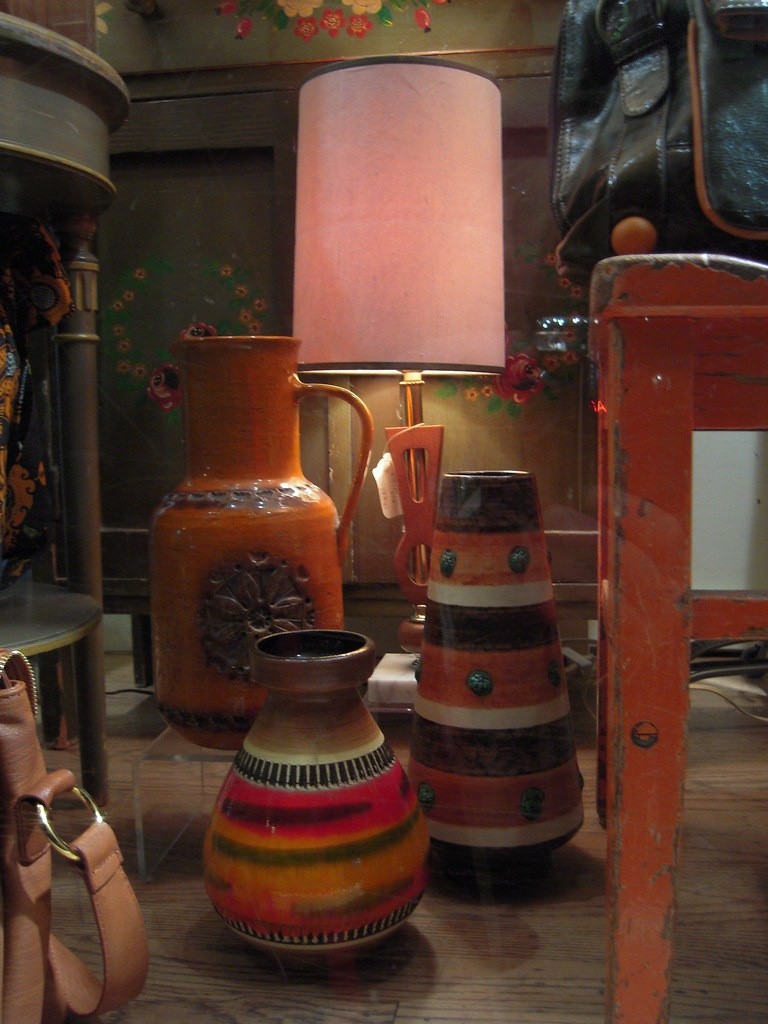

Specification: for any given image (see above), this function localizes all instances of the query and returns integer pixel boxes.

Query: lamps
[293,57,508,716]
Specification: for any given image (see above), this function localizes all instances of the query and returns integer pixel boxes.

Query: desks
[0,9,128,810]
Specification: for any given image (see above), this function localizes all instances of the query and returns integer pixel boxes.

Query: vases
[411,471,586,894]
[200,630,431,970]
[148,333,375,751]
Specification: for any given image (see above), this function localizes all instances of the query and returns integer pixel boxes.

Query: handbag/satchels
[0,647,149,1024]
[546,0,768,289]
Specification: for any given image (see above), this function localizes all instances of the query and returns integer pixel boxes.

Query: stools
[588,251,768,1024]
